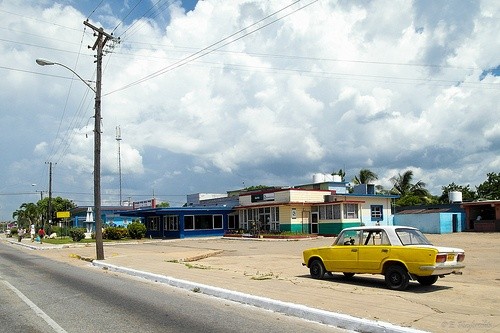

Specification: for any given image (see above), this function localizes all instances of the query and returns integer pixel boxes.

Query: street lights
[35,58,105,260]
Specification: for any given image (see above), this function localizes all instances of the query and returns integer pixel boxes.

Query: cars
[302,226,466,290]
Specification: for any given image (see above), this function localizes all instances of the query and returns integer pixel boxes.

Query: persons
[18,227,24,242]
[7,228,11,238]
[376,219,382,238]
[29,222,36,242]
[259,219,262,231]
[38,227,45,244]
